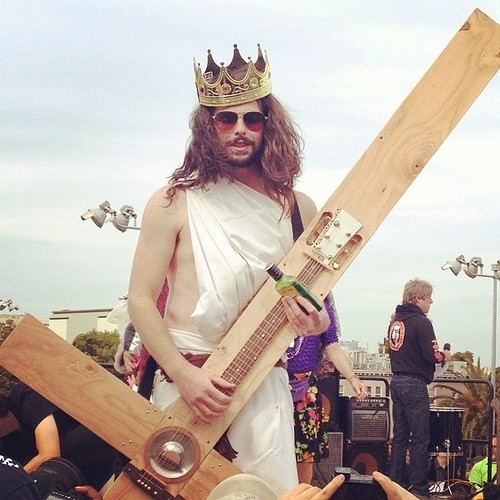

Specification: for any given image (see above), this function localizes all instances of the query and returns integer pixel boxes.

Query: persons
[285,292,368,484]
[0,382,125,474]
[429,451,456,483]
[386,278,451,498]
[0,455,103,500]
[126,40,333,500]
[469,436,499,490]
[109,297,153,400]
[281,471,420,500]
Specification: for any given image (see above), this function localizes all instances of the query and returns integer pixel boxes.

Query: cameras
[329,466,388,500]
[28,456,93,500]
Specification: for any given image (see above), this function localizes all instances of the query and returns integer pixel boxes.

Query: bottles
[264,261,322,317]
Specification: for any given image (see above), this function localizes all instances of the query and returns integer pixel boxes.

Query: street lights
[439,255,500,406]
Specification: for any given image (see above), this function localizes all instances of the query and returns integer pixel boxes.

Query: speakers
[312,396,391,489]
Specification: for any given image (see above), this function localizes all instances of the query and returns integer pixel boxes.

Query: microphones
[441,342,451,367]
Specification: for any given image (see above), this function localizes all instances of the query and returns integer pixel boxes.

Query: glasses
[209,110,269,133]
